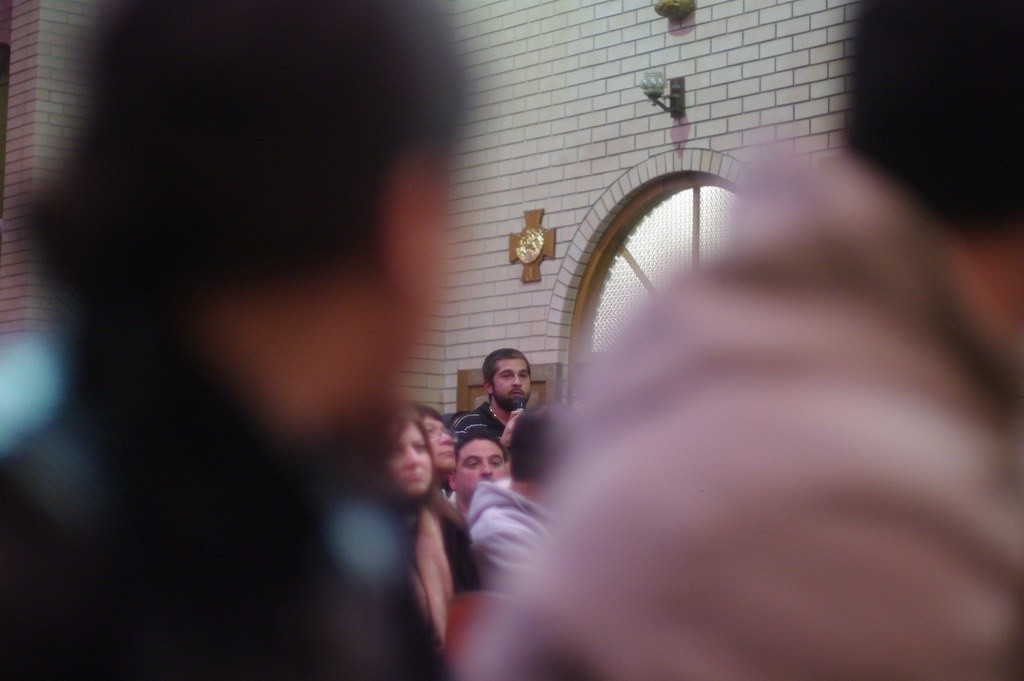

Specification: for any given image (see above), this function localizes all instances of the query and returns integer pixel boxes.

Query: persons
[470,0,1024,681]
[0,0,452,681]
[377,347,570,654]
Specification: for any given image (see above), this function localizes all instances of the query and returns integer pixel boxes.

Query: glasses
[423,422,455,442]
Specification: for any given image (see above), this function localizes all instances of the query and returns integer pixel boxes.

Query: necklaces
[489,406,506,425]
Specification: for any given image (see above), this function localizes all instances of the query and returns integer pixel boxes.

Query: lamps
[642,72,686,118]
